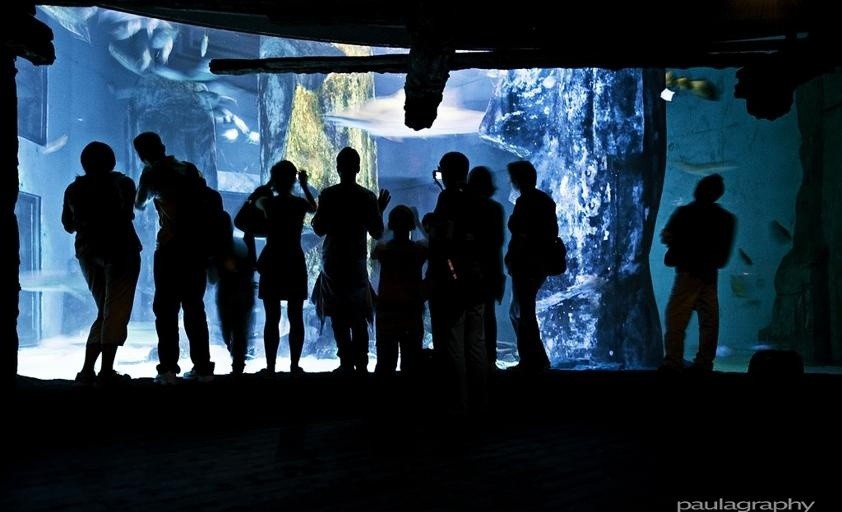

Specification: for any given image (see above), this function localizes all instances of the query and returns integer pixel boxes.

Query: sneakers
[97,371,130,384]
[507,359,551,373]
[183,362,215,382]
[153,367,176,385]
[73,372,96,385]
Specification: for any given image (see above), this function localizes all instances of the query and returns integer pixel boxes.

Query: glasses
[438,166,444,171]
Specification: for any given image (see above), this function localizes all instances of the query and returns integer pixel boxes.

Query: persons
[62,141,142,379]
[371,205,428,369]
[466,165,506,370]
[660,174,738,371]
[505,162,567,369]
[209,210,256,373]
[422,152,474,370]
[133,132,232,376]
[233,161,318,373]
[312,147,392,373]
